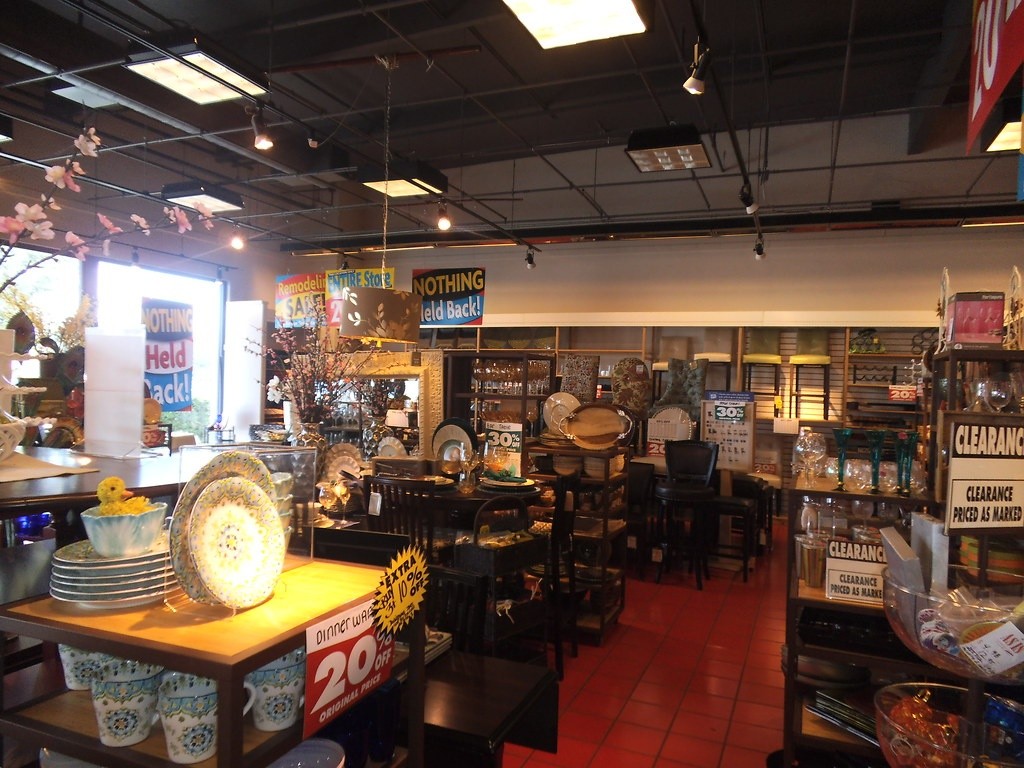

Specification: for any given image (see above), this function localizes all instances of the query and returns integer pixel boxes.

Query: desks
[290,500,547,655]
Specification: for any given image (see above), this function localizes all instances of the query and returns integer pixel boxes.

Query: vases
[289,423,328,495]
[40,359,58,377]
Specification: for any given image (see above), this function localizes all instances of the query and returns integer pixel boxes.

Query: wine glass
[317,481,350,526]
[473,362,548,395]
[480,406,539,440]
[796,429,927,546]
[460,443,508,484]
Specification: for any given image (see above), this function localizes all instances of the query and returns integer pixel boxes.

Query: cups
[54,643,306,764]
[796,535,829,587]
[963,372,1024,414]
[458,473,476,494]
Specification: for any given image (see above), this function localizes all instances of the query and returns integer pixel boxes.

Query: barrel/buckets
[267,738,346,768]
[40,748,102,768]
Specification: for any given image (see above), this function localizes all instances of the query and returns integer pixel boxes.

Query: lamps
[0,0,1024,343]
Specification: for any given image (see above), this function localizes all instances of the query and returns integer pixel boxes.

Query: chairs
[367,438,773,677]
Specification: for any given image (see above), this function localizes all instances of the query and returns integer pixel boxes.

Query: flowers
[0,123,229,296]
[242,289,410,423]
[1,271,98,359]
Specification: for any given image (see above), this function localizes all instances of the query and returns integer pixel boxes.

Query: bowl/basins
[882,565,1023,685]
[298,503,322,519]
[552,454,625,479]
[875,683,1023,767]
[80,501,168,558]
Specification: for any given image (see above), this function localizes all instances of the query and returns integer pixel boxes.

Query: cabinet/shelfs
[478,440,626,648]
[780,328,1024,768]
[0,445,454,768]
[443,351,557,451]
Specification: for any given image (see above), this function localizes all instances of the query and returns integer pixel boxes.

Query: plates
[653,407,692,443]
[324,443,363,481]
[543,391,582,436]
[377,437,407,457]
[430,417,478,475]
[49,450,287,611]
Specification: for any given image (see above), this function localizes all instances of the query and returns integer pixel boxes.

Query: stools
[651,352,832,421]
